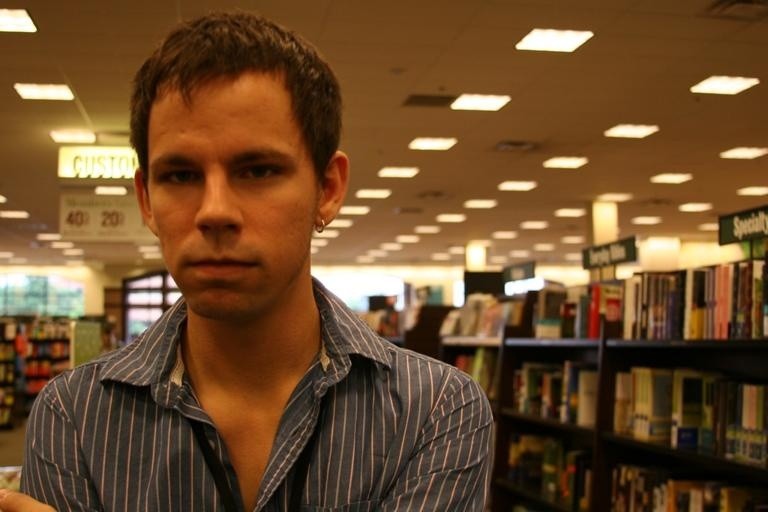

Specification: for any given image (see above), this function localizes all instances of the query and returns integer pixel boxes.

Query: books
[0,318,70,428]
[437,257,766,511]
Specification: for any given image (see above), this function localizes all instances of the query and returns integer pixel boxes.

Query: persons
[0,11,496,511]
[104,321,118,350]
